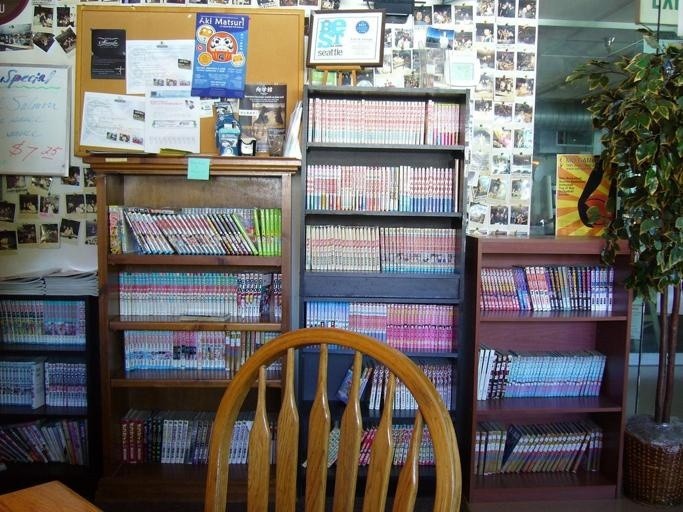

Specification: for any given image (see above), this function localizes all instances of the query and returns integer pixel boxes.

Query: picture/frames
[308,10,384,67]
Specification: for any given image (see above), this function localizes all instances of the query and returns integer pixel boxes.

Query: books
[480,264,614,312]
[1,244,101,468]
[119,271,283,317]
[477,344,607,400]
[109,205,283,258]
[307,158,460,212]
[336,357,452,411]
[239,84,286,150]
[308,99,460,146]
[475,420,604,476]
[305,224,457,273]
[119,406,281,466]
[301,418,436,468]
[306,301,454,353]
[124,331,283,371]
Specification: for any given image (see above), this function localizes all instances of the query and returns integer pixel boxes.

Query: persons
[463,100,533,236]
[2,165,98,250]
[382,1,536,97]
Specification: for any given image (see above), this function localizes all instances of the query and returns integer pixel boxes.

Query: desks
[1,481,103,512]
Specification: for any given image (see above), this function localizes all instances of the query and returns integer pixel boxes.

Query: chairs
[204,326,464,512]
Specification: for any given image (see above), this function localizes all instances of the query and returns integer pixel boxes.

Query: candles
[566,39,682,511]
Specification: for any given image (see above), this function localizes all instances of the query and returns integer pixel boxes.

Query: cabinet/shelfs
[1,294,97,468]
[301,84,469,498]
[465,234,639,503]
[84,152,299,504]
